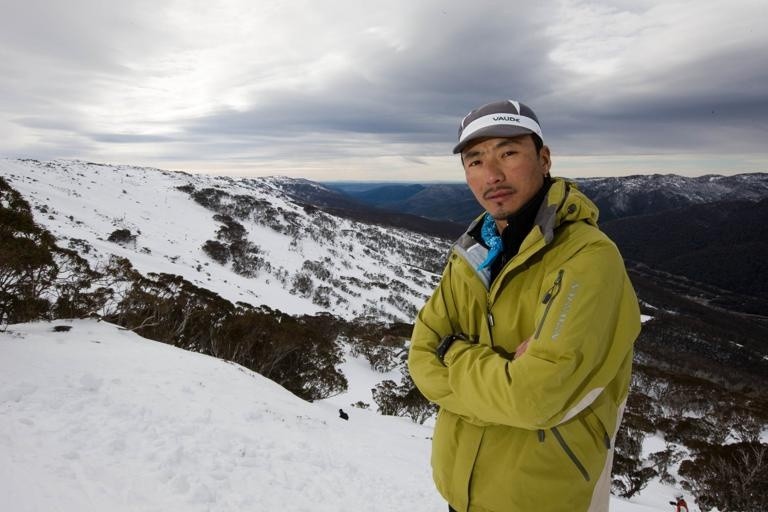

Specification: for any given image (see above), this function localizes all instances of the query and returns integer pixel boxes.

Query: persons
[408,100,641,512]
[676,495,689,512]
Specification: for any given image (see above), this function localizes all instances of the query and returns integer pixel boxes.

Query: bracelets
[435,334,465,368]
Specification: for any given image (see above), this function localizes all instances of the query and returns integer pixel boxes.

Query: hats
[452,99,543,153]
